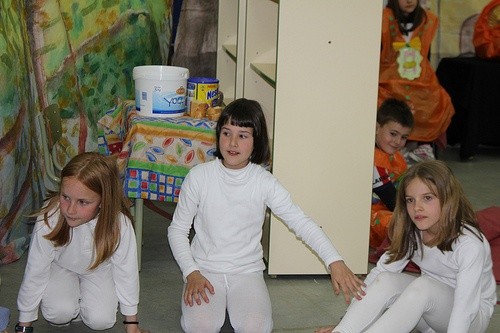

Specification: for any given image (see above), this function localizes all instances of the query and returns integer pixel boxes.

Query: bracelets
[123,321,139,325]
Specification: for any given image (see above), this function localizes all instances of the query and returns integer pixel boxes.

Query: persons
[167,98,367,333]
[377,0,454,164]
[15,152,141,333]
[315,160,497,333]
[368,99,408,255]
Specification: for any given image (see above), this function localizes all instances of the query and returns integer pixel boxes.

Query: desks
[95,100,219,272]
[436,56,500,156]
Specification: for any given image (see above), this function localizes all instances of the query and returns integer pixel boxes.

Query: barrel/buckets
[133,65,190,118]
[186,77,220,117]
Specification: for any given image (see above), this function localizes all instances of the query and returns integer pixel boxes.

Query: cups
[190,100,206,119]
[207,107,222,122]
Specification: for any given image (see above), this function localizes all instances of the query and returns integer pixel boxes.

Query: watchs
[15,323,33,333]
